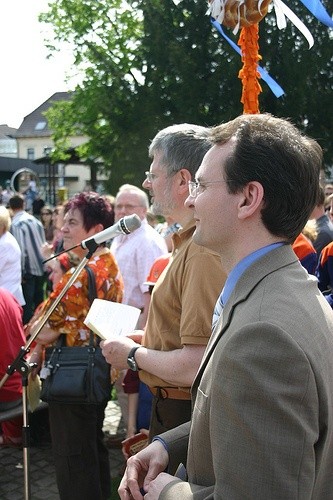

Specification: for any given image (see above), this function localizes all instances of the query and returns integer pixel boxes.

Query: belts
[149,385,193,402]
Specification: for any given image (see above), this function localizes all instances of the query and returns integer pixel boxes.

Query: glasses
[145,171,176,182]
[188,180,224,197]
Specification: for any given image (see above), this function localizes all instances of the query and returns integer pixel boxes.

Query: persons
[8,196,44,339]
[293,165,333,309]
[99,123,216,500]
[0,287,31,446]
[0,205,26,318]
[25,190,116,500]
[30,192,64,258]
[118,115,333,500]
[110,183,183,449]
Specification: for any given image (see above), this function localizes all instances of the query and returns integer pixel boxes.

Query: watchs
[127,346,143,371]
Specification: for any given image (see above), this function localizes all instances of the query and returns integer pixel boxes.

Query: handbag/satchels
[38,342,111,406]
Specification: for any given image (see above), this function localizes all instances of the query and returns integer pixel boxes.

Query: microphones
[80,213,141,249]
